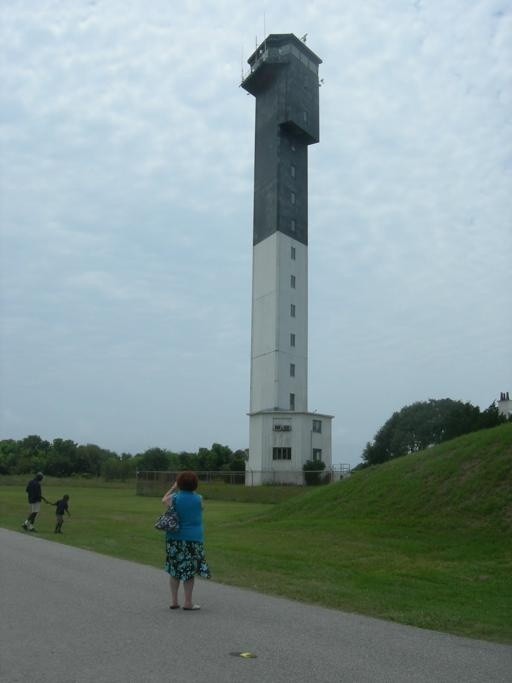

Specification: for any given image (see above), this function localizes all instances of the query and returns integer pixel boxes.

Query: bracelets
[169,487,177,492]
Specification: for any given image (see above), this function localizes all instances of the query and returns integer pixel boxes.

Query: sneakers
[21,523,28,531]
[29,527,37,533]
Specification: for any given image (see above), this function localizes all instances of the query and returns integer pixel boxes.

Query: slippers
[182,604,199,610]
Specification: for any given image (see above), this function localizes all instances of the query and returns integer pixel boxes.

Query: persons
[20,472,49,532]
[49,492,73,535]
[158,468,211,611]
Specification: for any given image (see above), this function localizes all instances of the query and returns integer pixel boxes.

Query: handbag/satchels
[154,507,181,534]
